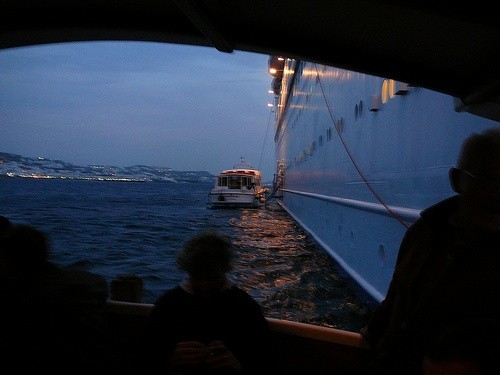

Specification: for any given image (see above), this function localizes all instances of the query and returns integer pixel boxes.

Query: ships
[272,44,499,303]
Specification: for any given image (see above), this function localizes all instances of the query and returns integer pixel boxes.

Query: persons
[148,229,273,375]
[359,128,500,375]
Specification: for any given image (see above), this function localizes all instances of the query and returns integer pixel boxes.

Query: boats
[207,157,264,210]
